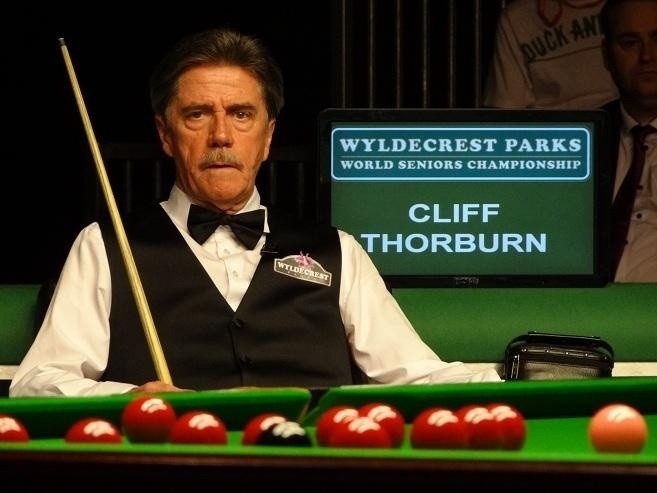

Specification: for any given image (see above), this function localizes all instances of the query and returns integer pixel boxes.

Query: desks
[0,372,657,492]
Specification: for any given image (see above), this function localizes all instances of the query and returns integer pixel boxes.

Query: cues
[59,37,172,386]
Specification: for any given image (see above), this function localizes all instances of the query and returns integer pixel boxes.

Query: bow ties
[188,204,265,250]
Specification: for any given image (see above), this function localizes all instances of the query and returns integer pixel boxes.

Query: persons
[595,1,657,284]
[485,0,621,109]
[7,25,504,397]
